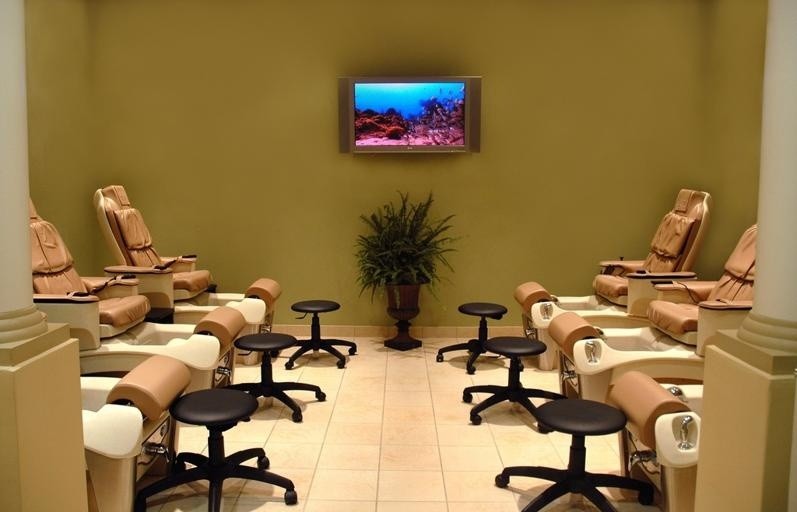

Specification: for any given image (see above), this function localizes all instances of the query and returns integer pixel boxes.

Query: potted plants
[351,189,455,351]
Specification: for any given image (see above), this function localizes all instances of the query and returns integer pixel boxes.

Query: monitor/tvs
[338,76,482,155]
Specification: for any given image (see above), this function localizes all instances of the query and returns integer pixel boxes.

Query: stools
[463,337,568,434]
[134,388,297,512]
[222,333,326,423]
[270,299,357,369]
[494,399,654,512]
[436,302,508,374]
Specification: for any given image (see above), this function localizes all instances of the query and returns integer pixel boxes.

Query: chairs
[549,224,757,404]
[29,196,247,393]
[605,371,704,512]
[92,185,282,366]
[514,187,712,371]
[81,355,192,512]
